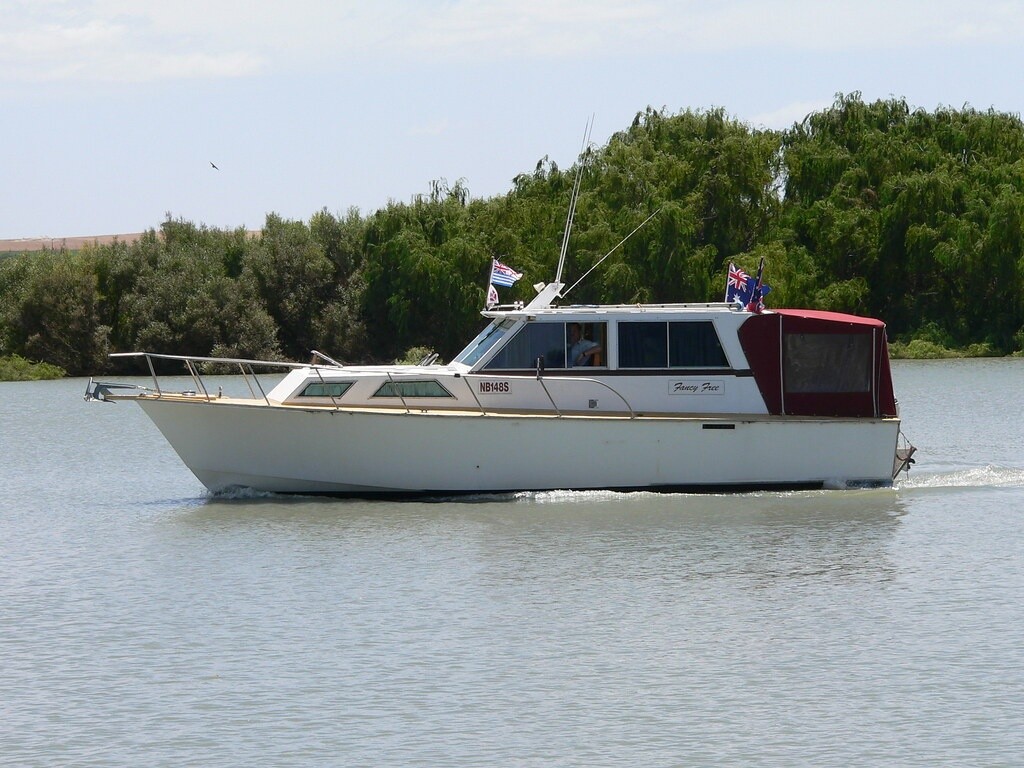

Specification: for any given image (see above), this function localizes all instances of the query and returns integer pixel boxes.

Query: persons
[536,323,602,367]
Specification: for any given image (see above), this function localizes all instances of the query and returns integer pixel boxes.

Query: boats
[87,114,918,500]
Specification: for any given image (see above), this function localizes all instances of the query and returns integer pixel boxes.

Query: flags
[724,260,770,315]
[487,261,522,305]
